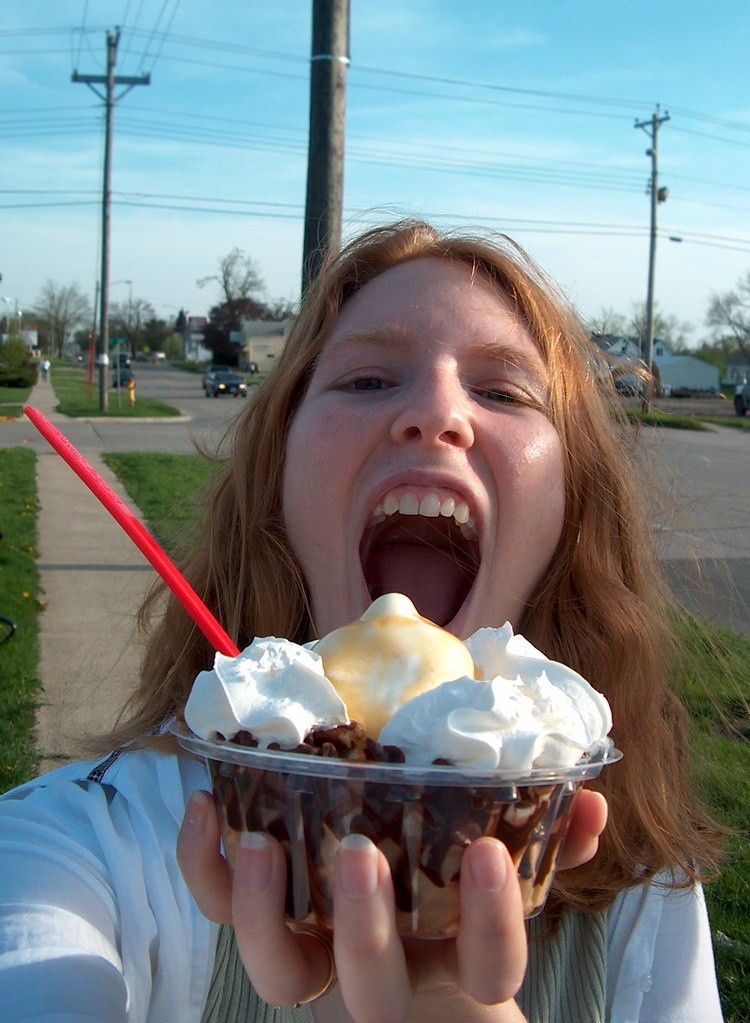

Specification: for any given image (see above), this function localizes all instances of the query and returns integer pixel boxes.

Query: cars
[734,377,749,417]
[205,372,248,399]
[26,345,43,359]
[112,368,135,388]
[202,367,232,390]
[616,379,672,397]
[110,353,131,369]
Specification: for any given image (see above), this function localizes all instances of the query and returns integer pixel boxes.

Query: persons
[40,358,51,380]
[0,221,725,1023]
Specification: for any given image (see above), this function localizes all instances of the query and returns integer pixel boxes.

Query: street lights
[17,310,22,337]
[2,297,11,334]
[88,278,131,383]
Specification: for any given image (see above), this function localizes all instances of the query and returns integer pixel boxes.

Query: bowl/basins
[161,709,624,942]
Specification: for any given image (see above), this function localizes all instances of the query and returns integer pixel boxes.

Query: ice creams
[180,591,616,944]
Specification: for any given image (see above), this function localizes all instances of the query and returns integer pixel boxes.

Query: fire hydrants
[128,380,136,407]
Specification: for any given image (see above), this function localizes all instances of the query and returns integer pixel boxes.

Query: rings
[291,928,337,1009]
